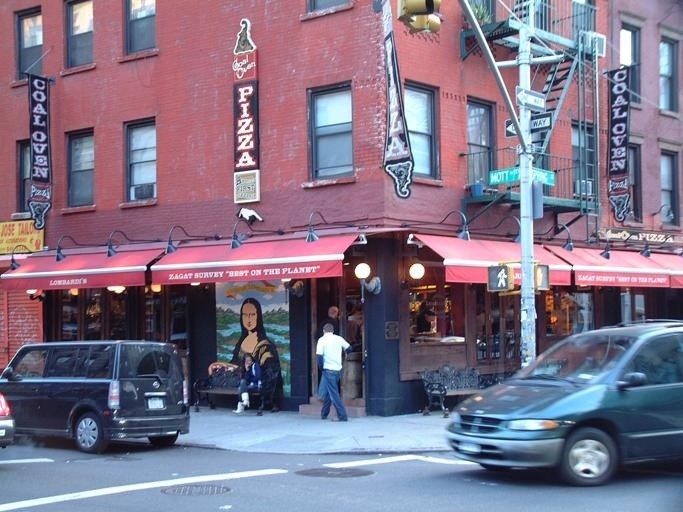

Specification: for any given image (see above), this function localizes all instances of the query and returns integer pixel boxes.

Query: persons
[313,322,354,423]
[635,337,678,387]
[315,305,339,402]
[409,310,436,343]
[230,353,261,415]
[204,296,283,398]
[589,345,609,372]
[254,344,283,416]
[343,301,357,321]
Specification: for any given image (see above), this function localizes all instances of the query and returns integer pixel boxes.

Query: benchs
[193,367,279,416]
[415,364,502,418]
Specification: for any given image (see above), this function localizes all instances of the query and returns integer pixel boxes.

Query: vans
[443,318,682,487]
[0,339,190,454]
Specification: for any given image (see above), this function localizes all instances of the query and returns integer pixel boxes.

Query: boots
[232,392,249,415]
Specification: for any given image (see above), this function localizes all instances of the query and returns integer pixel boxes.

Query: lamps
[354,262,382,305]
[231,217,285,249]
[55,235,108,261]
[165,225,224,254]
[26,289,45,302]
[190,282,212,291]
[8,244,34,269]
[436,203,675,259]
[281,278,304,297]
[400,262,426,292]
[305,212,330,244]
[107,230,163,257]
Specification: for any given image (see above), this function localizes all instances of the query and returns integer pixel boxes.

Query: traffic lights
[398,0,440,34]
[486,265,513,291]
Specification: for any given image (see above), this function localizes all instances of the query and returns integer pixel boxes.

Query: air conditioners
[586,30,608,58]
